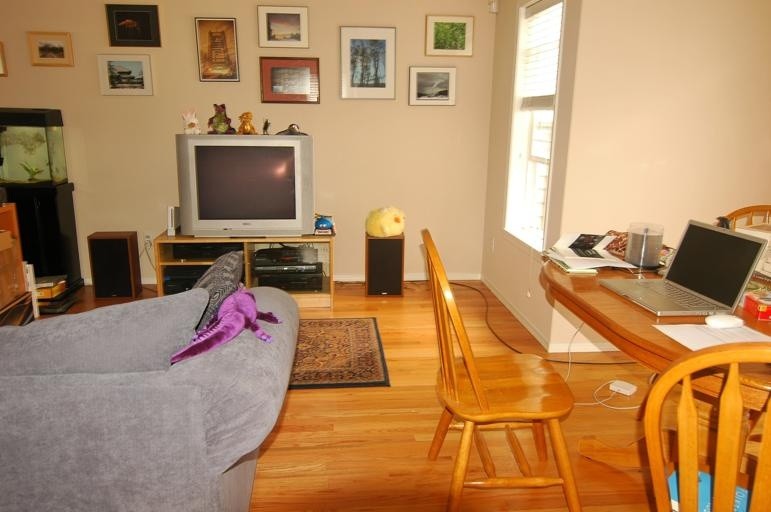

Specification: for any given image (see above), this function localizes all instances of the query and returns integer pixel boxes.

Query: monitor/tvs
[176,133,315,237]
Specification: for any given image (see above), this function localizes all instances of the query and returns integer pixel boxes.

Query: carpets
[289,316,392,387]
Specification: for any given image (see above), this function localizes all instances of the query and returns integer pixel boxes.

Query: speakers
[365,232,404,296]
[88,231,143,298]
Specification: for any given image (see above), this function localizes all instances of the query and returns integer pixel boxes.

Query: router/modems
[168,206,179,236]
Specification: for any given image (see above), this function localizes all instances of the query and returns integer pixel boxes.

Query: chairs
[413,223,584,512]
[1,285,299,512]
[642,341,771,511]
[719,202,771,233]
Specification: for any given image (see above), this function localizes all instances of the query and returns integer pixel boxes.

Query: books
[36,275,68,288]
[546,231,639,275]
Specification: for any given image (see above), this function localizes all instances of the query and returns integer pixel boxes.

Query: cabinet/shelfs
[0,181,86,302]
[157,228,334,313]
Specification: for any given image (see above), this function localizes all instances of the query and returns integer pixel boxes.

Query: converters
[610,380,637,396]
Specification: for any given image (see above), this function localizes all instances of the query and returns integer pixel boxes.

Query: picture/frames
[258,55,321,105]
[0,42,8,77]
[25,27,76,70]
[256,5,310,49]
[408,66,457,106]
[194,17,240,83]
[104,1,162,48]
[340,25,396,101]
[424,15,474,56]
[94,52,154,97]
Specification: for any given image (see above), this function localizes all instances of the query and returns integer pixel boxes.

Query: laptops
[599,219,769,317]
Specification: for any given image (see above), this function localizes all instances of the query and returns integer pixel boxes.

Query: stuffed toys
[168,280,279,365]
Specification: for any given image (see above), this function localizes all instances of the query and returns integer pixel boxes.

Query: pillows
[2,287,213,376]
[192,252,245,329]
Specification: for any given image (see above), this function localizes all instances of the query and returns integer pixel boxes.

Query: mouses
[704,315,744,329]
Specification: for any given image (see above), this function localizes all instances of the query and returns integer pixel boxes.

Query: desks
[539,246,771,413]
